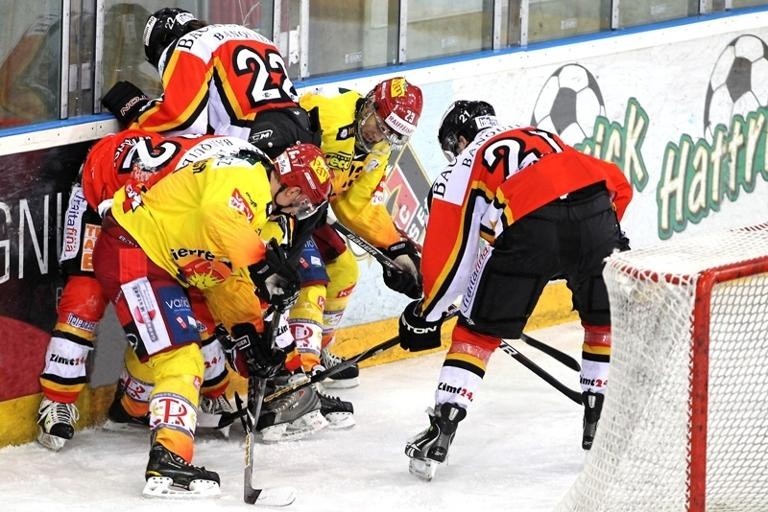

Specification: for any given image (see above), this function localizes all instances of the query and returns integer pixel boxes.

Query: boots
[582,393,604,450]
[405,402,467,464]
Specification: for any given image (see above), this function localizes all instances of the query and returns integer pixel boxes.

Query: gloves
[248,238,304,313]
[399,301,444,352]
[232,321,287,378]
[101,79,160,128]
[380,240,424,298]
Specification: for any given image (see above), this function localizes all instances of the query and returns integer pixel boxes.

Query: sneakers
[200,393,235,417]
[257,349,358,432]
[108,378,154,426]
[145,431,219,490]
[35,395,77,441]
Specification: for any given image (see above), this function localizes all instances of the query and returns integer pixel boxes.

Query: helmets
[140,6,198,69]
[273,142,332,220]
[370,78,423,147]
[437,101,499,155]
[103,4,149,71]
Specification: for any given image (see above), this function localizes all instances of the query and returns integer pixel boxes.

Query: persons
[100,8,321,430]
[36,128,286,439]
[92,143,333,491]
[398,101,633,461]
[287,77,424,422]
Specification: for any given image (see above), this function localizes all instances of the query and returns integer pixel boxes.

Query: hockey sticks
[197,309,461,428]
[339,220,585,404]
[244,291,294,508]
